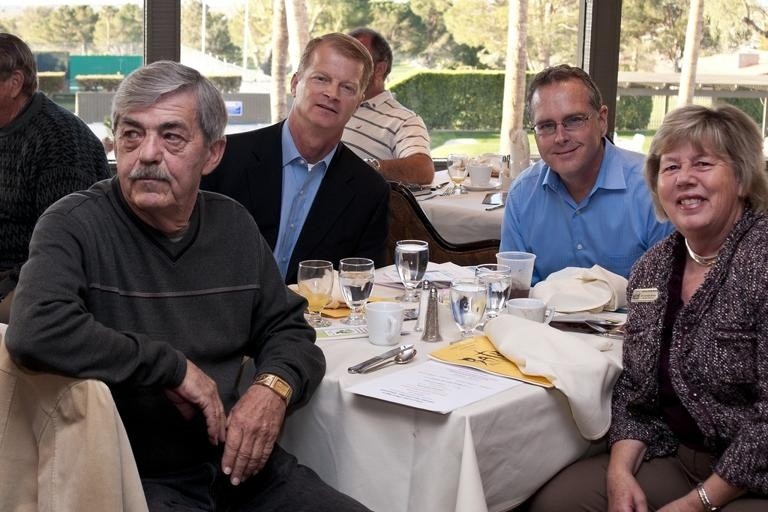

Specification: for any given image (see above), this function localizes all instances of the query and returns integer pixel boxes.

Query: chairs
[386,181,498,266]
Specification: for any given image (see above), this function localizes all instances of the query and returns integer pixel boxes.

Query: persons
[0,31,110,320]
[536,106,767,512]
[4,61,371,510]
[499,63,676,293]
[198,32,391,285]
[341,29,434,188]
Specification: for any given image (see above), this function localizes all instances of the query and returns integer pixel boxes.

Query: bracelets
[694,483,714,507]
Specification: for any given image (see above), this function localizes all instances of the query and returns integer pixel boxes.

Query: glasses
[530,107,598,136]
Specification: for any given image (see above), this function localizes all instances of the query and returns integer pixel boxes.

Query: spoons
[359,348,417,370]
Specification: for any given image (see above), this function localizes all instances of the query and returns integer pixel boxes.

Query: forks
[415,185,456,202]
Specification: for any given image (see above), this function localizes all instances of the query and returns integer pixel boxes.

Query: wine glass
[446,154,470,197]
[339,257,375,327]
[297,259,335,329]
[448,278,488,344]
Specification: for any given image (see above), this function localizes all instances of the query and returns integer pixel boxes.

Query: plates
[461,180,502,191]
[534,276,613,314]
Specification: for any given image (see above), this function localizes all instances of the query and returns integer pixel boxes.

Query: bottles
[499,155,512,190]
[415,278,443,344]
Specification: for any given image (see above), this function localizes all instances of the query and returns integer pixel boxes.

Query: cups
[365,301,405,346]
[505,297,555,324]
[468,164,493,186]
[394,240,429,304]
[496,251,536,302]
[476,264,512,326]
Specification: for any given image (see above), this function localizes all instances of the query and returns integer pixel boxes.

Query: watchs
[252,373,294,406]
[363,156,381,174]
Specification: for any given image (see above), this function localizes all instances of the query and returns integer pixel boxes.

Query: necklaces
[682,236,731,267]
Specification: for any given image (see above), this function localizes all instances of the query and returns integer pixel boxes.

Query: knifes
[348,343,414,372]
